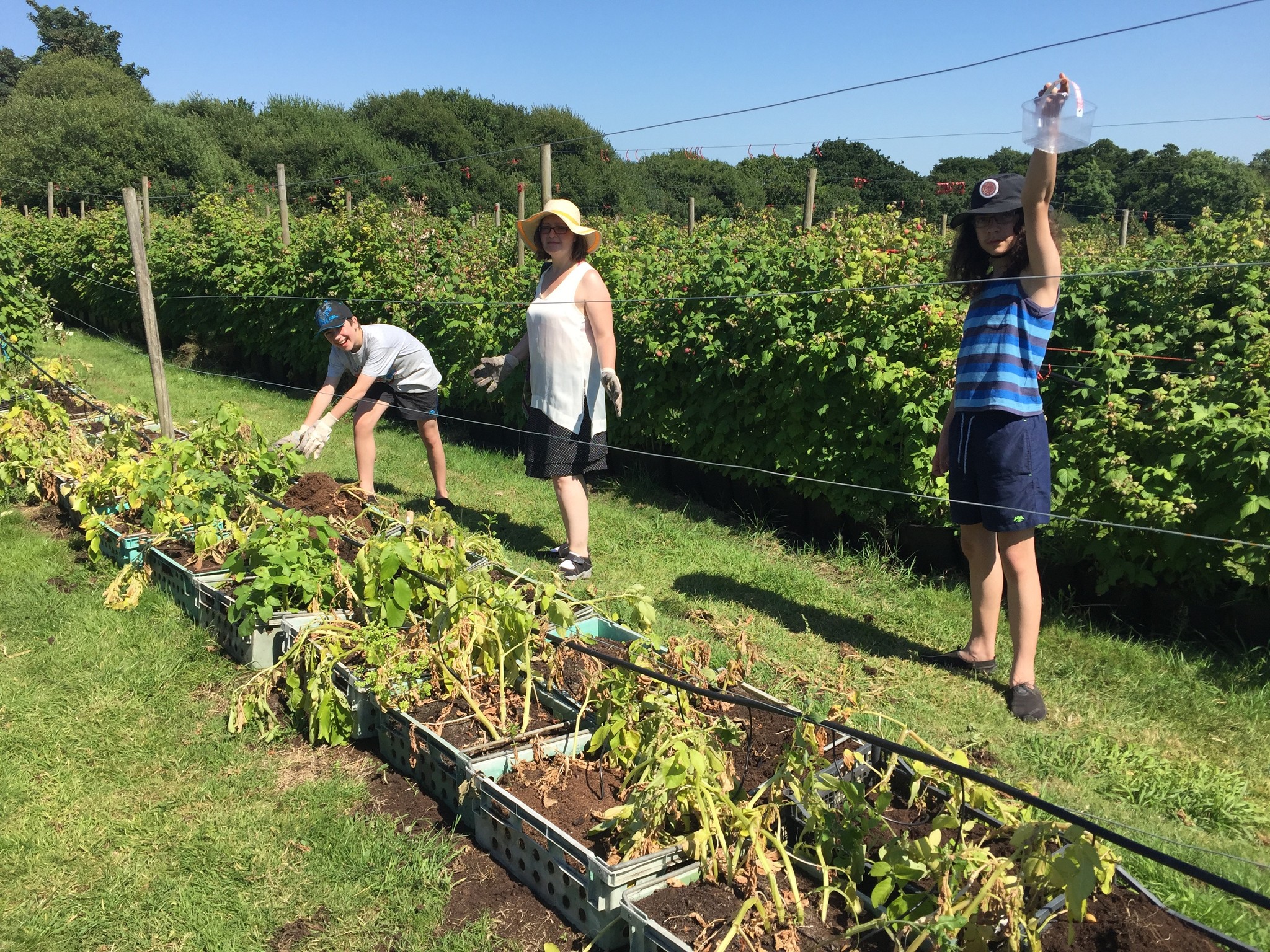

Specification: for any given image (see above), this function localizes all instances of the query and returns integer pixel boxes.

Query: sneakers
[557,551,592,580]
[532,542,569,562]
[921,644,998,672]
[1009,682,1045,724]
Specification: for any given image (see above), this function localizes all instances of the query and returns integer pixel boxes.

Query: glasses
[537,225,570,235]
[970,210,1018,228]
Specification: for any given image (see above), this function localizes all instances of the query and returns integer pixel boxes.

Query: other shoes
[361,496,377,509]
[433,497,455,512]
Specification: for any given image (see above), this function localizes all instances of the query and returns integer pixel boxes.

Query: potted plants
[0,311,1260,952]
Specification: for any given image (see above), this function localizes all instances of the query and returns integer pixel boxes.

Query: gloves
[268,423,309,458]
[469,354,519,394]
[601,368,622,418]
[298,412,340,460]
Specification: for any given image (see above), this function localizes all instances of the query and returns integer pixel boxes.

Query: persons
[932,71,1071,725]
[467,197,622,581]
[274,301,454,518]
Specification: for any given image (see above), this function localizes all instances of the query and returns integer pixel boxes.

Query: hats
[949,171,1024,231]
[315,300,354,333]
[515,199,602,255]
